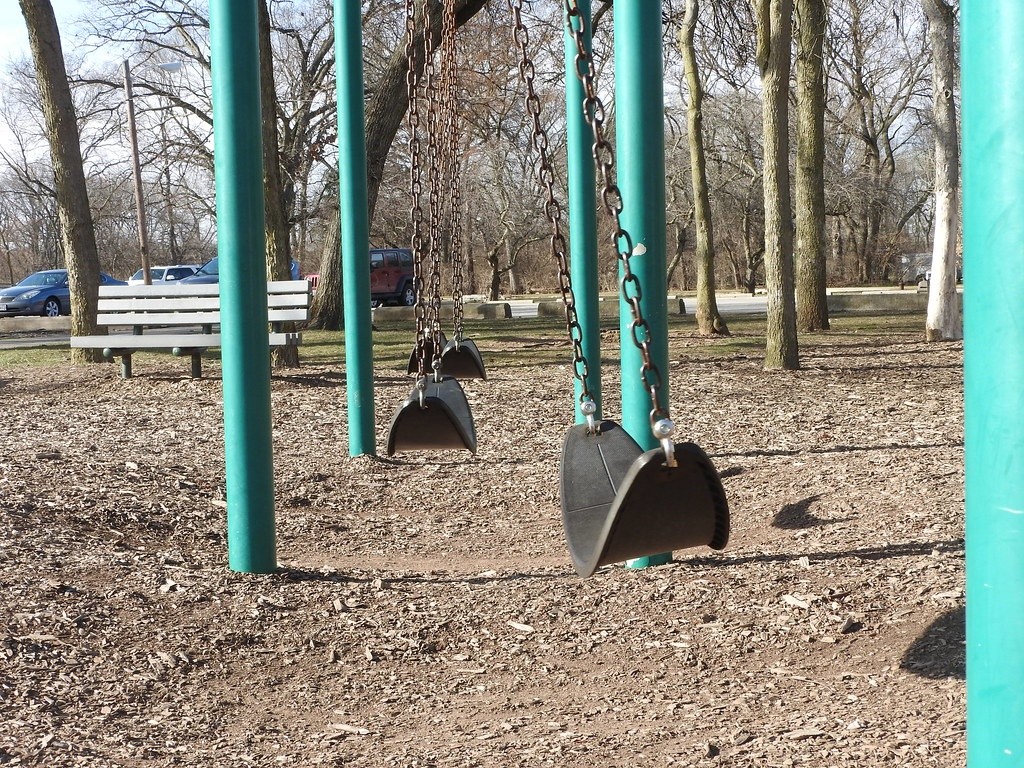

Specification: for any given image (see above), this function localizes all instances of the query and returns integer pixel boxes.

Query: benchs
[70,280,313,378]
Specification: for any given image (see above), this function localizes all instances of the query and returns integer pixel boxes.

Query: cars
[0,268,130,318]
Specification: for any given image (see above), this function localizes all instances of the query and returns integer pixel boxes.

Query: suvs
[172,256,301,284]
[127,265,202,286]
[303,248,414,307]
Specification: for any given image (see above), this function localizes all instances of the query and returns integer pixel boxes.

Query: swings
[381,0,490,455]
[505,1,730,580]
